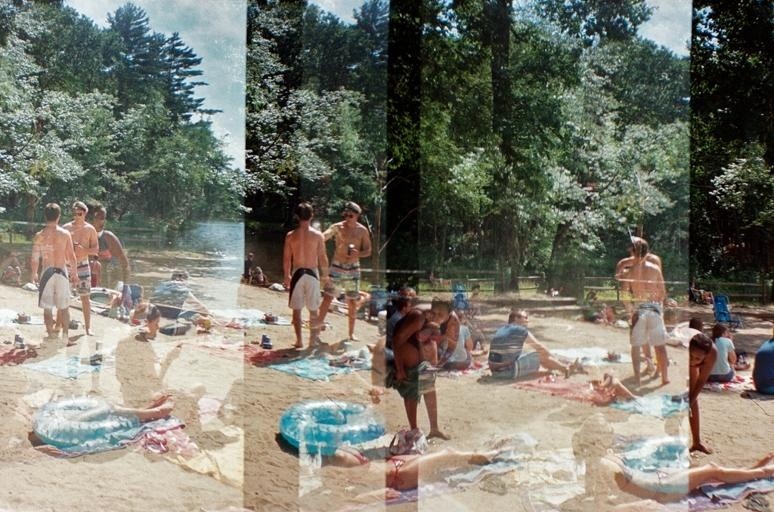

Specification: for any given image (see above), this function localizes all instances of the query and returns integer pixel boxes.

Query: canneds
[347,243,355,255]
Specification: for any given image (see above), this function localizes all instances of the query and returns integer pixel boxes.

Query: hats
[345,200,362,214]
[72,201,88,212]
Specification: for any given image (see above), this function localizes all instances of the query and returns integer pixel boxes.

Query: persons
[2,200,774,511]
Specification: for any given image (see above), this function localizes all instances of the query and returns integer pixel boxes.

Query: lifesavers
[33,398,139,455]
[278,400,385,458]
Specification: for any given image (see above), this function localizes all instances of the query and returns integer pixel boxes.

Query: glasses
[343,213,354,218]
[72,212,82,217]
